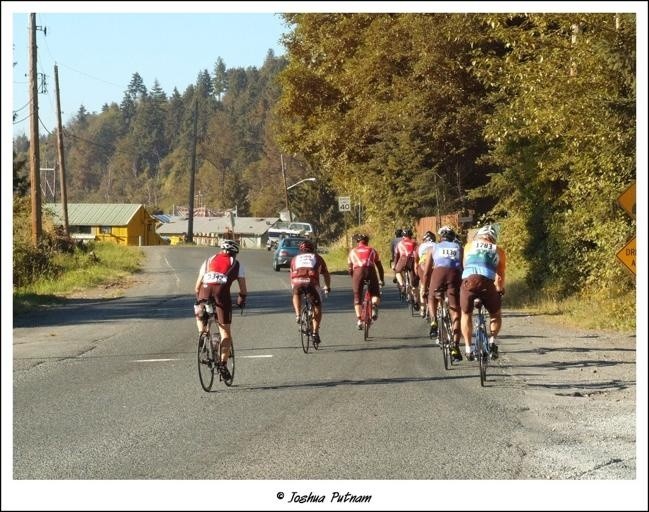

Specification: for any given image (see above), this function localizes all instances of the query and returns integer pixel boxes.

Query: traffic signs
[338,197,351,211]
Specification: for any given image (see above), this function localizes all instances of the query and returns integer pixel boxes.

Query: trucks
[267,222,317,251]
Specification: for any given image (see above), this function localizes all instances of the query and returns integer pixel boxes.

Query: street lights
[281,153,317,223]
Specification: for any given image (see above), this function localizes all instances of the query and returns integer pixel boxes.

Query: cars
[272,236,316,271]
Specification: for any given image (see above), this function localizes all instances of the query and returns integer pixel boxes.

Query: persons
[348,234,385,329]
[290,241,331,344]
[422,226,464,361]
[195,240,247,377]
[391,229,403,282]
[459,225,506,362]
[414,231,437,317]
[393,229,421,311]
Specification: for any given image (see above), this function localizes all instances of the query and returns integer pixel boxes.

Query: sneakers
[295,315,302,324]
[219,362,232,380]
[313,333,321,343]
[198,332,206,347]
[372,307,378,320]
[357,320,362,330]
[393,279,497,362]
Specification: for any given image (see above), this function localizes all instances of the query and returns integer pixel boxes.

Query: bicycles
[357,281,384,341]
[465,289,507,387]
[194,298,247,392]
[297,286,331,354]
[390,259,441,342]
[430,283,461,370]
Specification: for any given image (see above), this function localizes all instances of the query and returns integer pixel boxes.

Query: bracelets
[238,292,247,298]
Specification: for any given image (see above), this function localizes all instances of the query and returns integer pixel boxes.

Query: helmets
[355,234,368,242]
[221,240,239,253]
[394,225,497,242]
[300,241,314,252]
